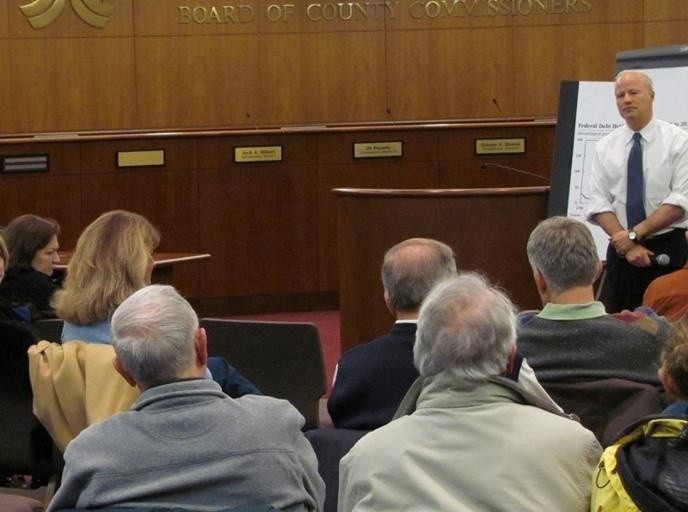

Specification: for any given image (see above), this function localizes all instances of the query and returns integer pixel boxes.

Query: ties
[626,133,646,244]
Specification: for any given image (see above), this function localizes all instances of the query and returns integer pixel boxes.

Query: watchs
[628,227,639,244]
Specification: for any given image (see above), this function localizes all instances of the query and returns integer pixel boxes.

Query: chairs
[200,319,327,434]
[0,318,52,488]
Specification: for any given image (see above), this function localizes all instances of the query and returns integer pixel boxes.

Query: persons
[0,233,55,491]
[643,229,688,322]
[1,216,62,320]
[591,322,687,512]
[584,68,688,313]
[337,267,604,512]
[49,283,326,512]
[516,216,664,447]
[328,236,460,428]
[50,208,262,400]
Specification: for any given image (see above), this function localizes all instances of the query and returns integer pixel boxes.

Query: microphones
[386,109,393,121]
[481,162,550,181]
[492,98,502,114]
[617,253,671,266]
[247,113,258,130]
